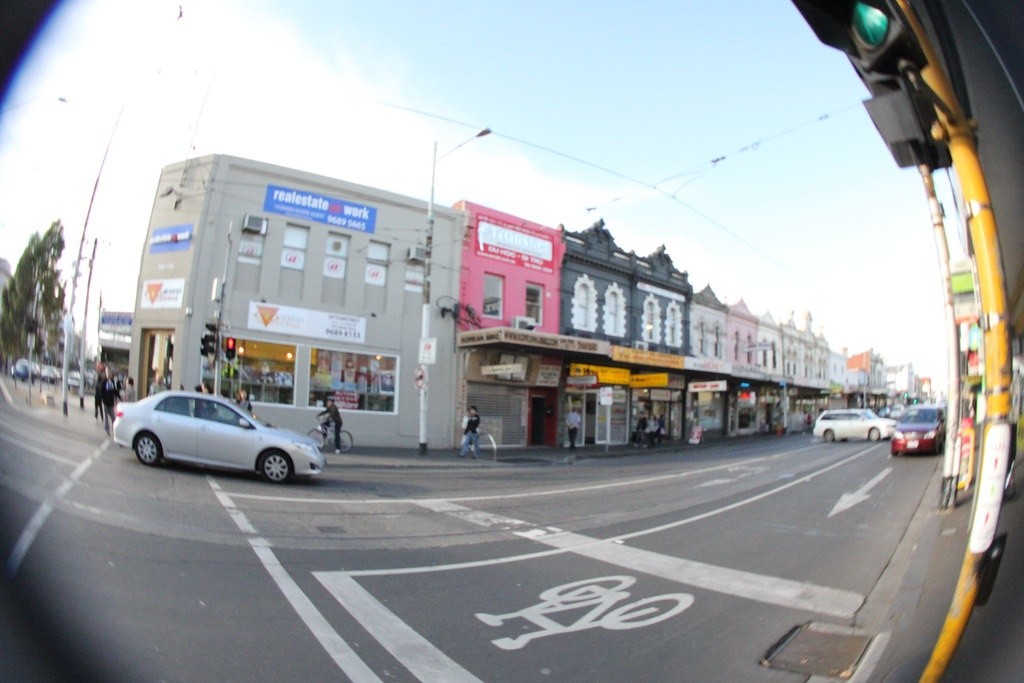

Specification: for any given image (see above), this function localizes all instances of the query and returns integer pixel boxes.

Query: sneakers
[335,449,341,455]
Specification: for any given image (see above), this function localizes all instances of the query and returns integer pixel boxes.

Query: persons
[656,413,666,445]
[633,411,648,448]
[645,414,660,449]
[460,407,476,452]
[91,364,254,448]
[806,413,813,431]
[457,405,481,459]
[565,405,581,451]
[317,395,344,454]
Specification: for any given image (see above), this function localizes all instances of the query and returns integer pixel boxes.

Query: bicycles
[309,416,353,453]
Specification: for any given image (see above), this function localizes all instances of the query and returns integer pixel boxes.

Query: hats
[326,396,336,401]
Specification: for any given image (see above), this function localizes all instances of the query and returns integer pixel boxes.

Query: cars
[11,359,80,391]
[113,391,325,484]
[891,408,946,455]
[813,408,898,442]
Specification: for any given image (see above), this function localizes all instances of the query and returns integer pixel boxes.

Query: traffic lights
[200,324,216,356]
[791,0,927,78]
[226,337,236,360]
